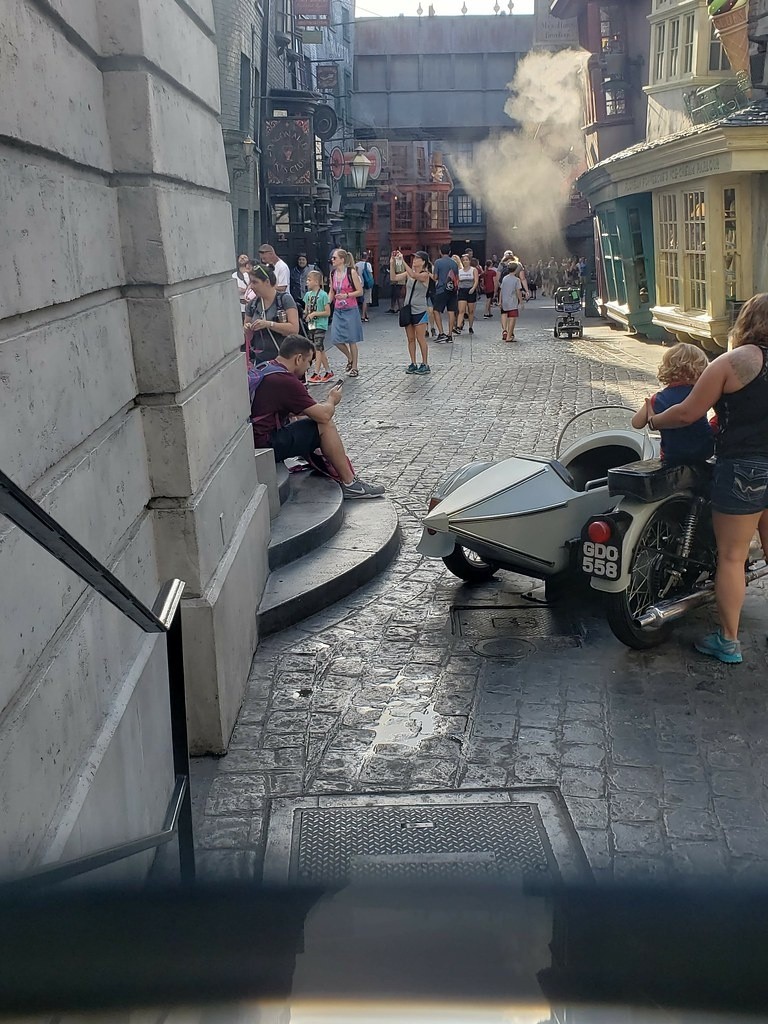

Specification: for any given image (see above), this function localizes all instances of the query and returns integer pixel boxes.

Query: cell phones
[332,379,344,390]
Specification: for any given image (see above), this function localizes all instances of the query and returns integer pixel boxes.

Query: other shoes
[361,313,371,322]
[452,326,463,334]
[384,307,401,315]
[469,327,474,334]
[345,360,359,377]
[483,313,494,318]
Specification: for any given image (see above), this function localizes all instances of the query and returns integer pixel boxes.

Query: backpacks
[331,266,365,307]
[247,360,292,431]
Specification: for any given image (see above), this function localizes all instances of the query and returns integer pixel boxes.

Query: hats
[410,250,430,261]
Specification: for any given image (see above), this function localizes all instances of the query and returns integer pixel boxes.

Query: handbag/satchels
[362,261,375,289]
[399,304,412,327]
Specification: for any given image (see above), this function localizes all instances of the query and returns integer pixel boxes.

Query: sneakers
[308,373,322,383]
[406,363,431,375]
[694,628,743,664]
[433,333,453,343]
[321,370,336,383]
[340,474,385,499]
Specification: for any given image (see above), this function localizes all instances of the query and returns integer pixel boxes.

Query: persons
[631,342,719,466]
[390,251,430,374]
[644,292,768,664]
[290,252,324,338]
[232,243,290,351]
[528,256,591,299]
[452,254,479,334]
[385,253,406,315]
[355,252,373,322]
[328,249,363,377]
[426,249,530,338]
[498,261,523,342]
[251,334,386,499]
[243,263,299,366]
[302,270,335,385]
[427,245,459,343]
[426,164,453,227]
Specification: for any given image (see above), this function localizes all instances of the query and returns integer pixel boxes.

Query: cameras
[339,300,347,306]
[392,251,399,257]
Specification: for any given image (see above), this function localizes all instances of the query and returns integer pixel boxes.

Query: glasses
[258,249,271,255]
[253,264,269,278]
[331,255,339,260]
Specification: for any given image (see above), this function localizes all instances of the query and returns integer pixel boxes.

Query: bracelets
[270,321,273,327]
[526,291,529,292]
[347,293,348,298]
[648,416,656,432]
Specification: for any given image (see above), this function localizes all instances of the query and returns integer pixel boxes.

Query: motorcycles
[417,405,768,650]
